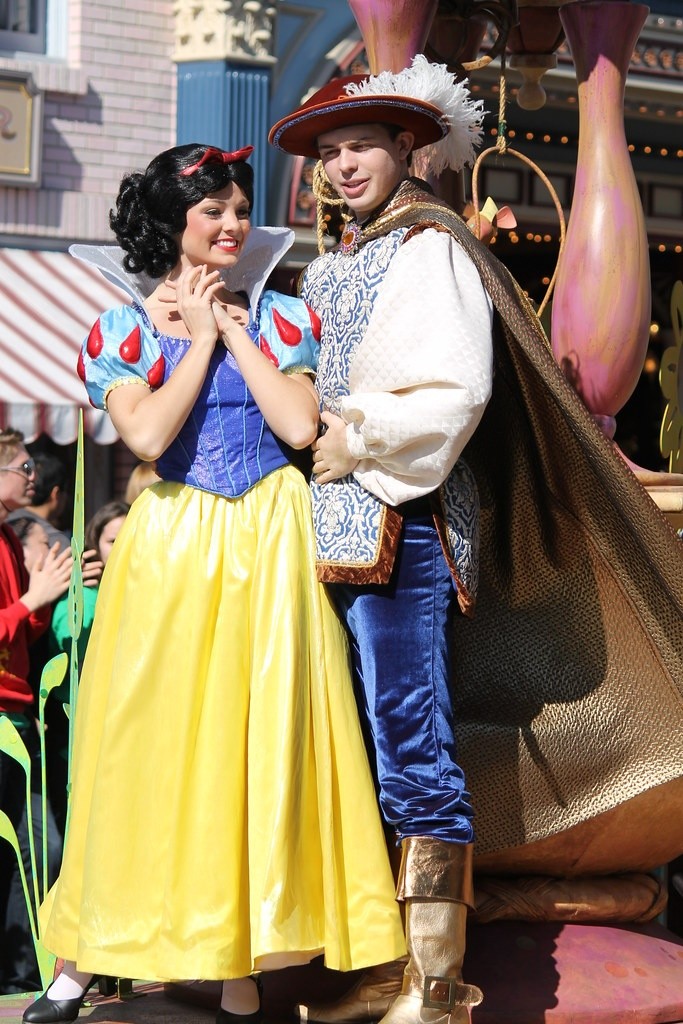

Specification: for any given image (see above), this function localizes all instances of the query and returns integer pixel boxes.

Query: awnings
[0,247,134,445]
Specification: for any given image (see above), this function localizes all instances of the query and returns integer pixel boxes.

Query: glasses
[1,457,33,475]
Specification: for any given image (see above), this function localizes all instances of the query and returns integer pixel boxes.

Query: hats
[268,54,492,178]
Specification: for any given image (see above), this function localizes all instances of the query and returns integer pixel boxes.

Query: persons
[0,425,164,996]
[20,142,410,1024]
[269,53,495,1024]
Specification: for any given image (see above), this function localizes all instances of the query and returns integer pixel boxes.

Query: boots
[374,837,484,1024]
[290,846,410,1024]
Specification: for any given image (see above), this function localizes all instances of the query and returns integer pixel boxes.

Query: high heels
[216,975,264,1024]
[21,973,117,1024]
